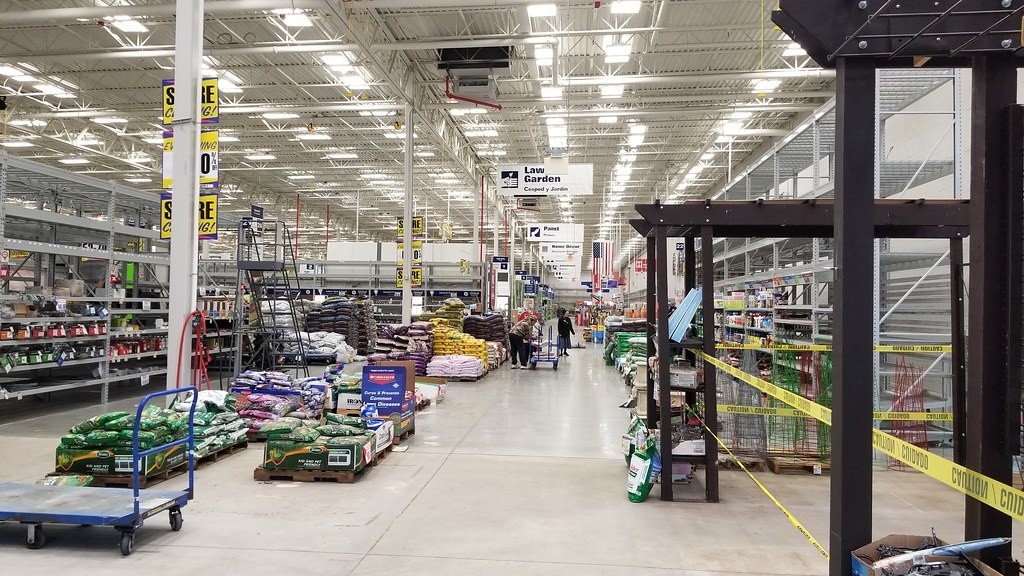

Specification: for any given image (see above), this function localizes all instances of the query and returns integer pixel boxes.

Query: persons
[572,309,587,348]
[509,315,538,370]
[558,308,575,356]
[523,322,541,365]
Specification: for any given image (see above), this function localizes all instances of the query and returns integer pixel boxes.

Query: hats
[530,315,538,322]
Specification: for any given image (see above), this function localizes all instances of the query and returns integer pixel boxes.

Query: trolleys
[0,386,198,555]
[270,350,337,366]
[529,324,560,371]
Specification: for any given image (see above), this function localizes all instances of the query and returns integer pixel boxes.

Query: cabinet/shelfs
[1,154,241,412]
[645,226,719,502]
[196,262,487,327]
[695,66,964,447]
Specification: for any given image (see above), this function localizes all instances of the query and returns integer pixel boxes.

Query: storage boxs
[263,433,376,471]
[656,392,685,409]
[370,417,393,454]
[635,390,647,416]
[670,368,702,388]
[56,438,188,477]
[672,461,696,474]
[361,360,415,436]
[336,384,361,417]
[415,377,448,399]
[851,534,1003,576]
[633,361,647,388]
[658,473,689,483]
[304,379,338,409]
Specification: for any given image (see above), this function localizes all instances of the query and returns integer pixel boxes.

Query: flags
[592,240,615,276]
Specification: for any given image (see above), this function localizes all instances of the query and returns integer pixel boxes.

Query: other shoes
[563,352,569,356]
[511,364,520,369]
[521,365,528,369]
[559,354,563,356]
